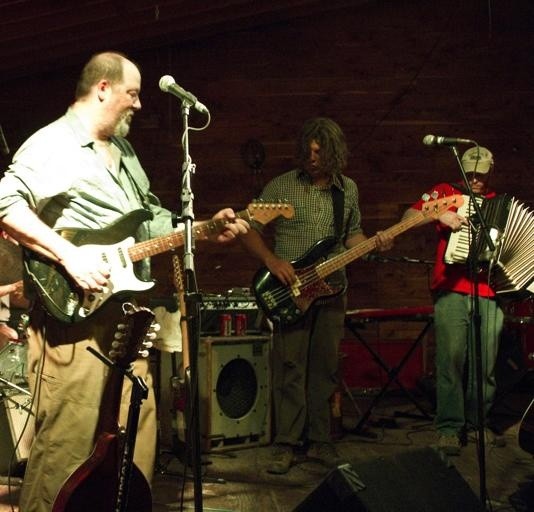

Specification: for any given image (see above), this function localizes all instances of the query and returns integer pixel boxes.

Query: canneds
[218,313,232,336]
[235,313,248,336]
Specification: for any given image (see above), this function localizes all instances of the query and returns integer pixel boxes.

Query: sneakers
[314,443,346,463]
[266,449,294,474]
[437,434,461,456]
[466,428,506,449]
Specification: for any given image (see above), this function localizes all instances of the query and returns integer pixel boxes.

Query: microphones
[159,74,206,113]
[422,134,472,148]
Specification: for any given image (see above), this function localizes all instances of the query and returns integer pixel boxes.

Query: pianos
[344,305,434,327]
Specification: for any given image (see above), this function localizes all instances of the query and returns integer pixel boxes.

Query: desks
[344,306,436,435]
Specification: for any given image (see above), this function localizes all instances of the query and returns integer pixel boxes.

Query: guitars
[49,302,161,510]
[253,188,464,331]
[21,197,296,324]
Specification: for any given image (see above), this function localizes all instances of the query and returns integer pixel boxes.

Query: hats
[460,146,495,176]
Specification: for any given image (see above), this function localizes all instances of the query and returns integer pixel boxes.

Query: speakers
[290,446,489,512]
[160,330,273,441]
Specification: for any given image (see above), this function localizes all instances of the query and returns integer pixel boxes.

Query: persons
[0,52,250,511]
[240,117,394,474]
[0,279,32,341]
[402,146,505,456]
